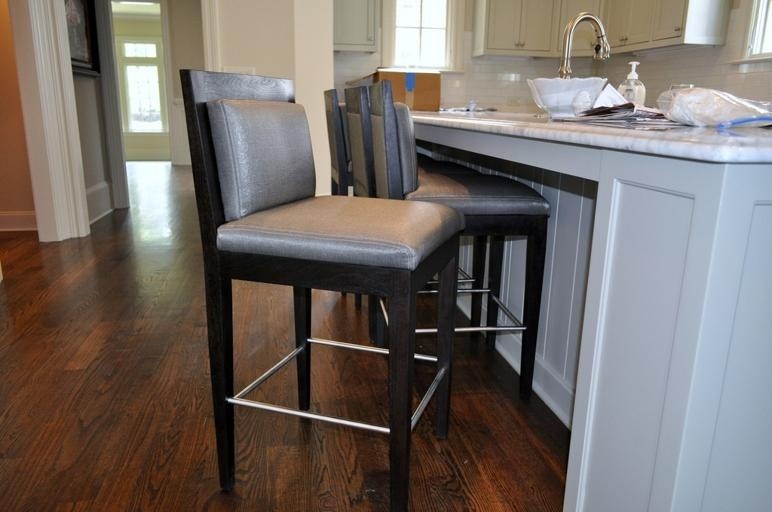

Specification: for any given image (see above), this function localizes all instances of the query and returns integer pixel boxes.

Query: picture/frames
[64,0,102,79]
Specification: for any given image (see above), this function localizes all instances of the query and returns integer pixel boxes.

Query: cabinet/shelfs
[472,0,733,57]
[333,1,379,55]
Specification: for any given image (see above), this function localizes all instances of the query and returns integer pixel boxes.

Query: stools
[324,78,549,400]
[177,69,464,510]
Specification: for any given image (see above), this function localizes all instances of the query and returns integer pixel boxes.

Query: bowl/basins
[524,75,608,121]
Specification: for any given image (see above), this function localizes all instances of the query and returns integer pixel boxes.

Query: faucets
[557,10,611,78]
